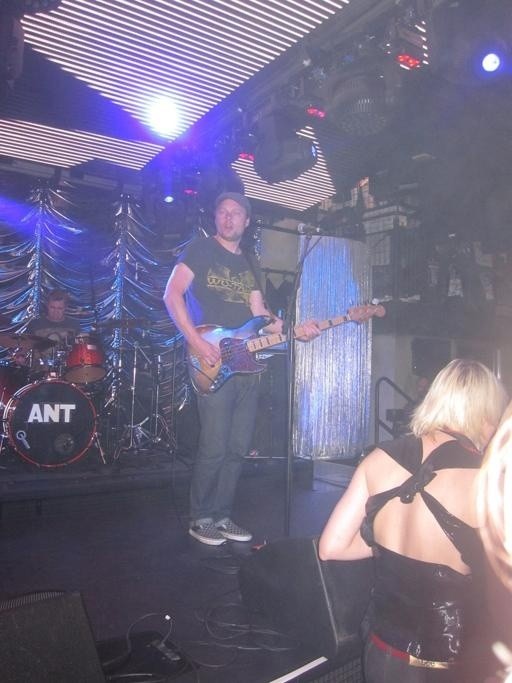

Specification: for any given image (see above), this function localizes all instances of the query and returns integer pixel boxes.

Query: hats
[215,191,251,215]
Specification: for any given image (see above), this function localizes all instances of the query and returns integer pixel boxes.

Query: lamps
[247,32,402,186]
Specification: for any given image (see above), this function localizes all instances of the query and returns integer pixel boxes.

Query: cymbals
[98,316,155,332]
[0,329,53,354]
[112,346,131,354]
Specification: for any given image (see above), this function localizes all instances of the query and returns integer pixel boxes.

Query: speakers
[236,535,375,661]
[0,589,106,683]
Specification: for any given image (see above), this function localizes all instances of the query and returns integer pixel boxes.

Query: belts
[368,630,462,671]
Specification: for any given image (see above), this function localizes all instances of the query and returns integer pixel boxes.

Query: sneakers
[217,519,253,542]
[188,522,227,546]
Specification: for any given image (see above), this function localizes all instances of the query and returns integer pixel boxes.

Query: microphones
[78,306,101,312]
[297,223,322,235]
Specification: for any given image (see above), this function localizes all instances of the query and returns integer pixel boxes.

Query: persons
[317,357,512,682]
[477,395,512,588]
[13,283,85,369]
[159,190,323,547]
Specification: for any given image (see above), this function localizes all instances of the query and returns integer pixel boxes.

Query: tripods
[99,336,178,464]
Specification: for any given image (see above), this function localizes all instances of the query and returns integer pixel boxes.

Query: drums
[0,363,29,409]
[64,332,108,386]
[4,380,97,469]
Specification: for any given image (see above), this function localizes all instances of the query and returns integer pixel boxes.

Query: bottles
[354,186,366,215]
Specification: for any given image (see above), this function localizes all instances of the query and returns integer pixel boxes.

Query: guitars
[183,300,401,399]
